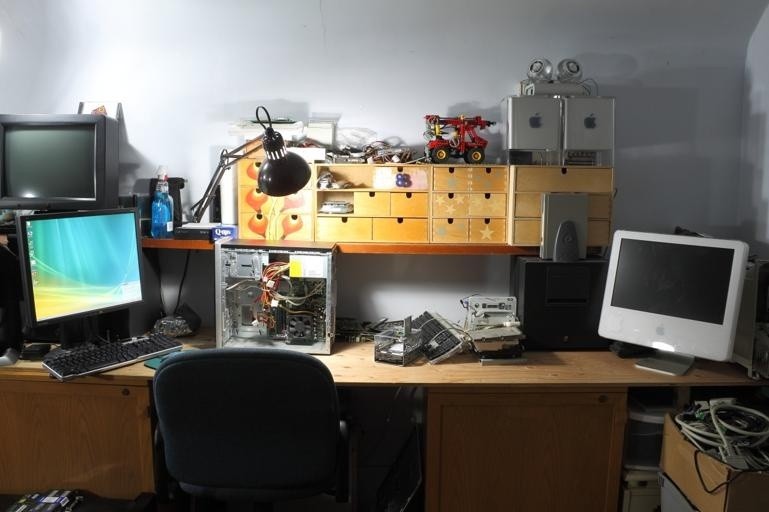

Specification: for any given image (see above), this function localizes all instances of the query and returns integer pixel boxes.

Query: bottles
[149,164,175,239]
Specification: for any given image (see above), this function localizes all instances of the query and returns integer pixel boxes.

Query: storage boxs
[663,408,768,511]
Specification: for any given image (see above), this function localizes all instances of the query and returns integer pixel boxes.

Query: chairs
[152,349,425,509]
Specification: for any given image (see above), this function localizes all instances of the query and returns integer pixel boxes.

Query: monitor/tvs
[0,114,117,212]
[15,208,148,357]
[596,228,749,376]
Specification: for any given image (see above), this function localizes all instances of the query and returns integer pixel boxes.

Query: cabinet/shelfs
[1,375,152,497]
[427,387,624,511]
[628,387,736,509]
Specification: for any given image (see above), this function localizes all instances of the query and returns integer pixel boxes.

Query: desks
[1,327,769,508]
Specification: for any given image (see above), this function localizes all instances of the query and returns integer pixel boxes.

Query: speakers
[510,256,609,351]
[555,57,583,85]
[526,55,553,83]
[66,248,160,344]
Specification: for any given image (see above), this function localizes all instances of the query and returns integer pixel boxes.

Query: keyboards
[41,331,183,382]
[411,311,466,363]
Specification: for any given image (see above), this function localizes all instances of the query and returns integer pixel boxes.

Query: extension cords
[708,396,737,409]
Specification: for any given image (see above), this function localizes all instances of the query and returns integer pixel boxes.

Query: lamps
[172,107,312,237]
